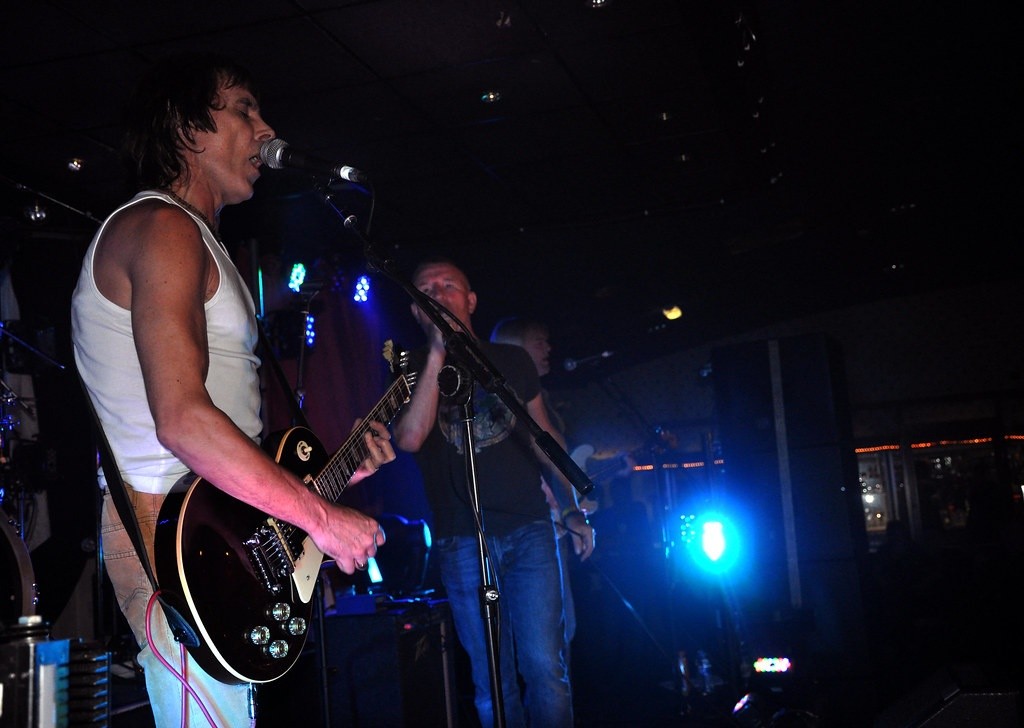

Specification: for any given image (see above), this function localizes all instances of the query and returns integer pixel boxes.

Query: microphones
[564,350,616,370]
[259,136,368,183]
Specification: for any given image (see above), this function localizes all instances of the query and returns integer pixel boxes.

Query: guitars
[156,357,421,685]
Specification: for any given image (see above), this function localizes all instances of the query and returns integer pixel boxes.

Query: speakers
[875,663,1024,728]
[256,612,405,728]
[712,331,880,681]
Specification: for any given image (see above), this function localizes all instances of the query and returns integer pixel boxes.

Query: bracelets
[561,512,585,520]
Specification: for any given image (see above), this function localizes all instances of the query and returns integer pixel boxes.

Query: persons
[253,257,393,728]
[382,264,597,728]
[599,472,1024,728]
[489,315,596,647]
[71,61,387,728]
